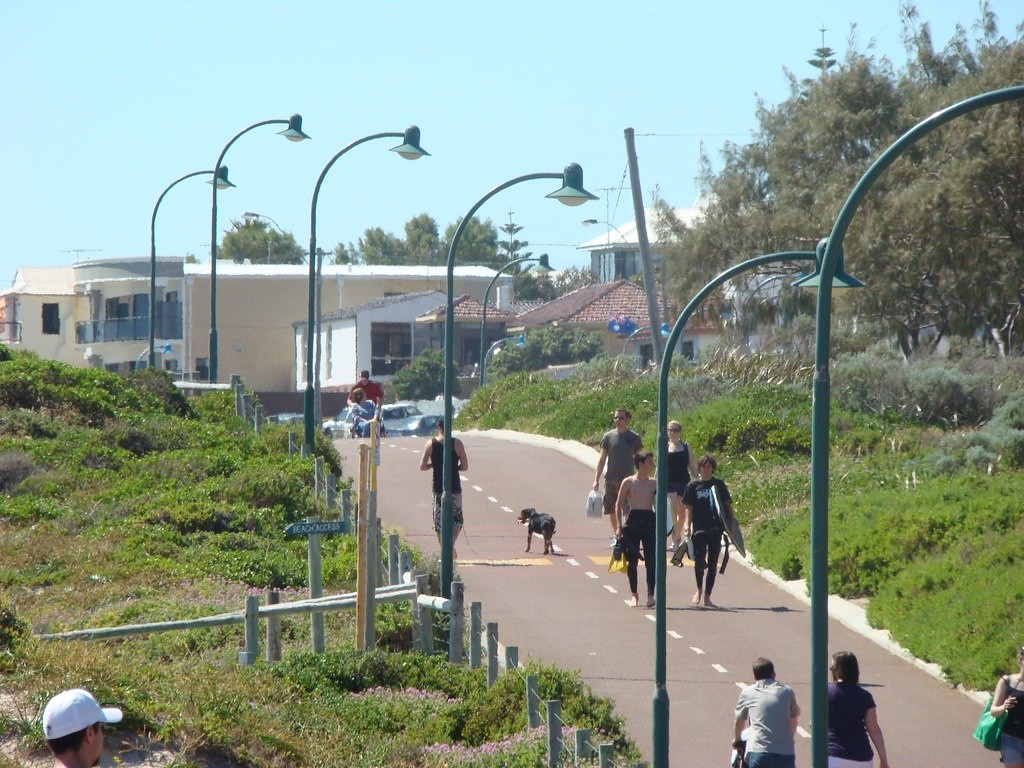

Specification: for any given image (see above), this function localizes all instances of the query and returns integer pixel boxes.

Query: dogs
[517,508,556,555]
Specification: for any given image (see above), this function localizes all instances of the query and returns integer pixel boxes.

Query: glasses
[666,425,683,432]
[614,415,626,421]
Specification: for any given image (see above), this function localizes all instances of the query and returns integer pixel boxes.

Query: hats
[42,688,123,740]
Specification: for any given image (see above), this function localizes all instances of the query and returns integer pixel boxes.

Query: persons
[732,657,802,768]
[653,421,700,550]
[420,421,468,562]
[41,688,122,768]
[593,409,643,539]
[990,648,1024,768]
[810,652,889,768]
[683,456,734,608]
[615,449,658,609]
[347,370,383,438]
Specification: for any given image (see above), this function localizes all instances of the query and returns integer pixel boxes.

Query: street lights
[134,343,175,371]
[439,162,599,596]
[480,252,556,389]
[648,236,870,768]
[208,112,310,387]
[484,333,528,386]
[304,124,431,458]
[147,163,237,369]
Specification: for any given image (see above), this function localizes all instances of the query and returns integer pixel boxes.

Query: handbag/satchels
[972,692,1009,752]
[585,488,603,518]
